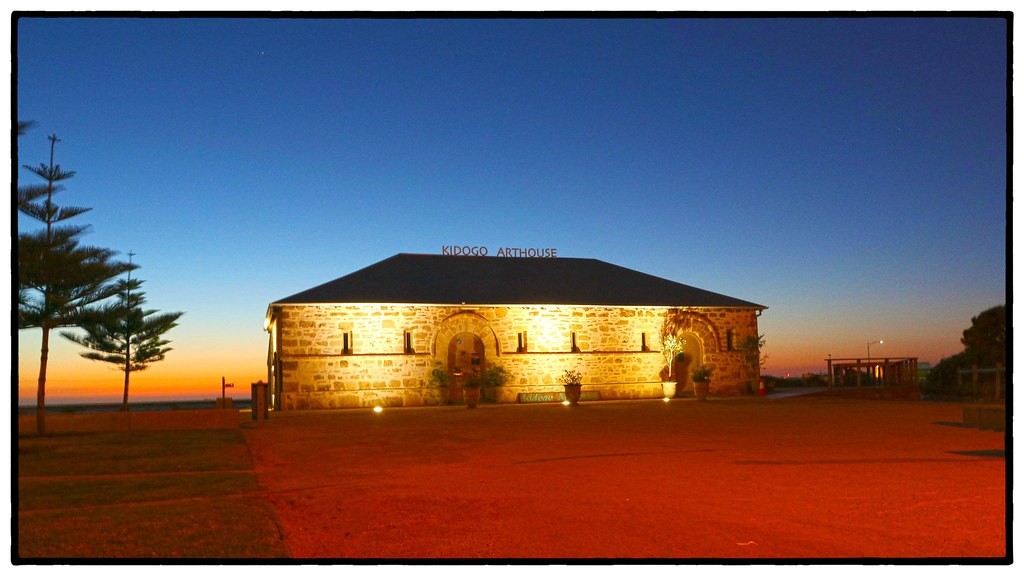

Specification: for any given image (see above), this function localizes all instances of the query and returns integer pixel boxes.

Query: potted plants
[744,334,769,397]
[691,370,711,400]
[463,377,482,408]
[484,365,512,402]
[659,334,685,399]
[432,364,451,405]
[560,369,584,404]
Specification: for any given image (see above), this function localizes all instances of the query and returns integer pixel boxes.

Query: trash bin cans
[252,383,269,420]
